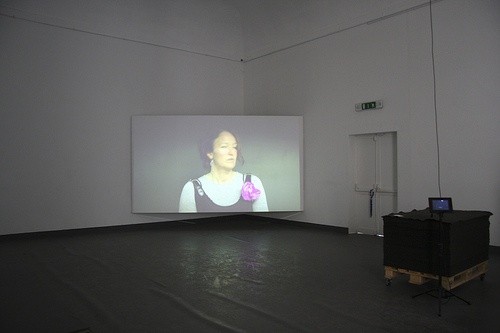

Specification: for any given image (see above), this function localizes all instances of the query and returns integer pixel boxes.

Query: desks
[380,205,494,297]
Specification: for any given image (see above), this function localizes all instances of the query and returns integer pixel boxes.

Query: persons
[178,124,270,212]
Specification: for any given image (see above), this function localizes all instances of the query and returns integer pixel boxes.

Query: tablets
[428,197,452,214]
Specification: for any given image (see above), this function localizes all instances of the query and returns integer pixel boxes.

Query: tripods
[409,216,471,317]
